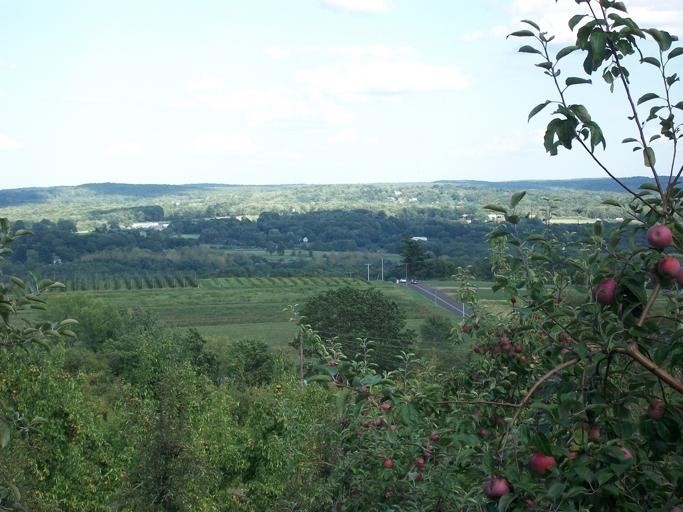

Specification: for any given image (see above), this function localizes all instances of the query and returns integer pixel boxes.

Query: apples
[327,223,683,512]
